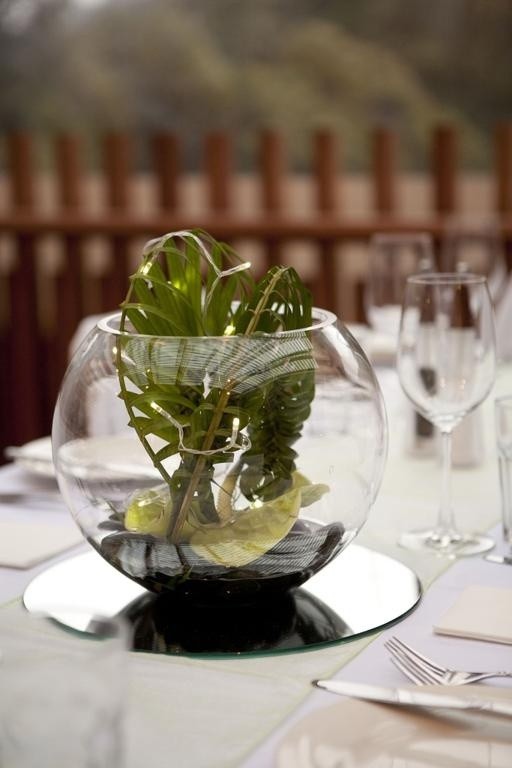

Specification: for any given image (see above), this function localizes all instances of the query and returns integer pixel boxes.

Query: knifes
[312,678,511,719]
[4,445,166,481]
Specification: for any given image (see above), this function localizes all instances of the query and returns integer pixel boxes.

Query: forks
[383,636,511,687]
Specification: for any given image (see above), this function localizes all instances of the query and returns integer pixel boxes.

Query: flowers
[84,224,336,566]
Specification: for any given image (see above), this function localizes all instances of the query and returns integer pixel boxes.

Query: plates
[16,436,166,484]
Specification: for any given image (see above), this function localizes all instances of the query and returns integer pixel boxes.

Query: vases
[15,301,427,662]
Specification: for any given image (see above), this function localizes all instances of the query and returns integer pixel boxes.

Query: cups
[362,232,436,336]
[438,241,508,308]
[492,396,510,567]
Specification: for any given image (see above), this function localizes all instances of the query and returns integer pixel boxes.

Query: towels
[3,514,83,576]
[435,580,512,651]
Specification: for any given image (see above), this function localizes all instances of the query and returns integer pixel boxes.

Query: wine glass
[397,273,496,559]
